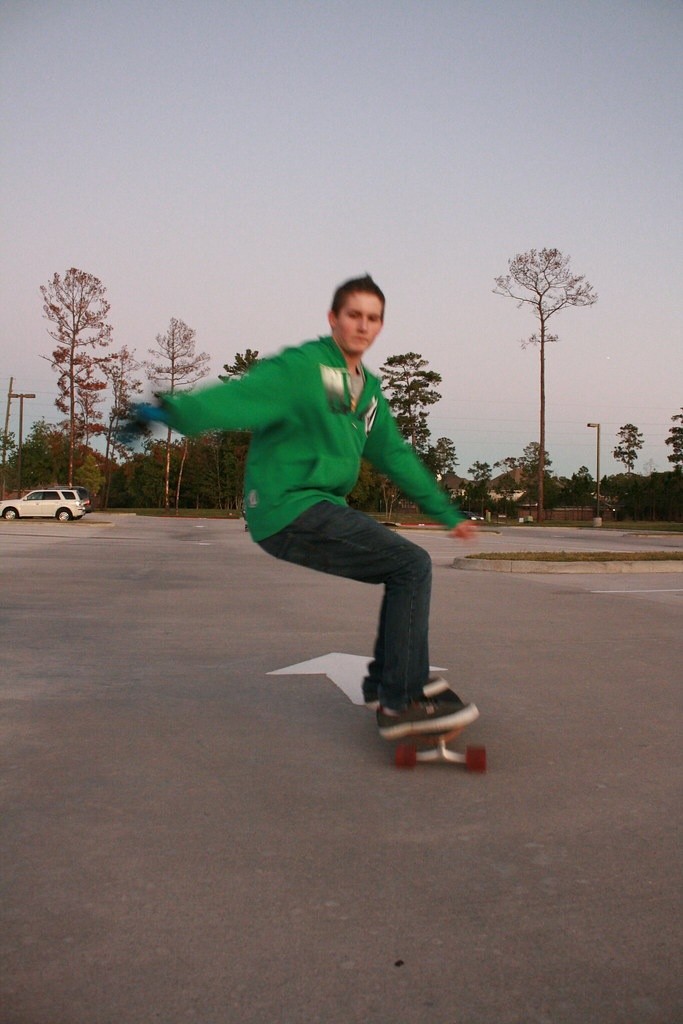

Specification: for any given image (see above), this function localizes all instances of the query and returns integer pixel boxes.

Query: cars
[459,511,484,520]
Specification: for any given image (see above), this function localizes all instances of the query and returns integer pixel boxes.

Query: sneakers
[362,675,450,710]
[376,701,480,743]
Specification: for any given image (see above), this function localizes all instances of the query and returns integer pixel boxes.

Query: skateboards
[374,681,487,773]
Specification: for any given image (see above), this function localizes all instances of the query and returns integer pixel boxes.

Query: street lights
[587,423,603,527]
[8,393,35,498]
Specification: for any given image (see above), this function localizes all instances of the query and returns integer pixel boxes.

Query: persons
[129,275,480,740]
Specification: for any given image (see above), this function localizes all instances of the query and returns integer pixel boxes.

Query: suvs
[0,490,86,522]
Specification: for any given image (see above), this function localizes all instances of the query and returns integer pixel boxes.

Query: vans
[45,486,91,513]
